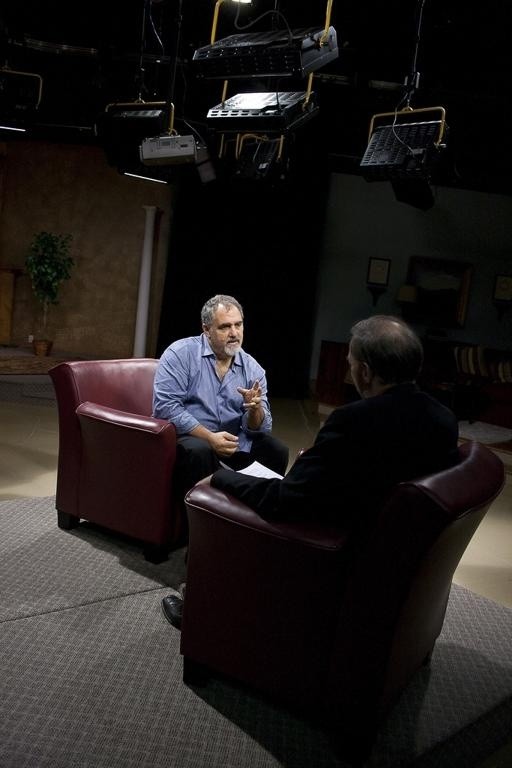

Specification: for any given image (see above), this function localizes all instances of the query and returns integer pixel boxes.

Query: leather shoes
[160,590,186,632]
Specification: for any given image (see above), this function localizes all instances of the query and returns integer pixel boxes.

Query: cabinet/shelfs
[314,339,512,429]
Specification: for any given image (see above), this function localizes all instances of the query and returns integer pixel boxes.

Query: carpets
[0,493,512,768]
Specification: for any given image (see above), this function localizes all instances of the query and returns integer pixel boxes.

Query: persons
[152,294,289,563]
[161,314,459,630]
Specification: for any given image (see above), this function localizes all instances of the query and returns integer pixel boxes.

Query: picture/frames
[365,256,391,286]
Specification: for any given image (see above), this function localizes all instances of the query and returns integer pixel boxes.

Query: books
[460,343,512,384]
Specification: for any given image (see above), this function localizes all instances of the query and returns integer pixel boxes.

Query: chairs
[180,441,506,763]
[47,358,189,566]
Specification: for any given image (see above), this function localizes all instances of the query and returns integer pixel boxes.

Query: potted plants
[22,231,80,357]
